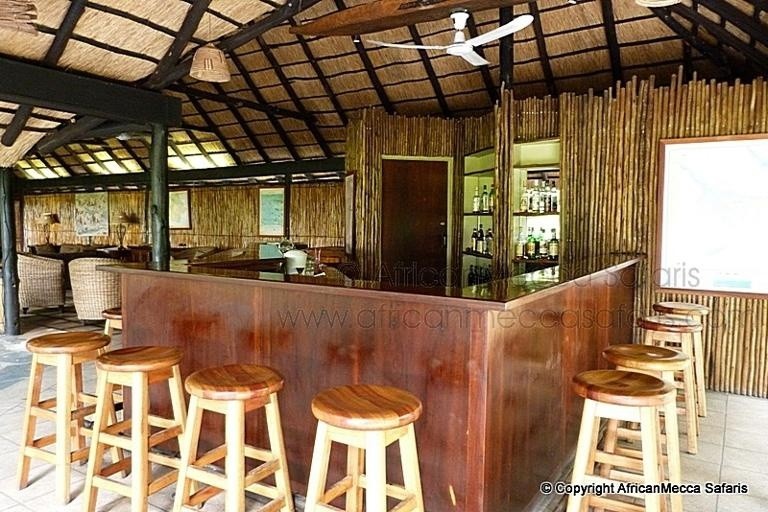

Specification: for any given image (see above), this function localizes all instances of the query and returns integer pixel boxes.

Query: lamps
[189,0,229,84]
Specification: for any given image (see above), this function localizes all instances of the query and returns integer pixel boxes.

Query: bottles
[472,180,559,213]
[468,264,493,287]
[472,224,559,260]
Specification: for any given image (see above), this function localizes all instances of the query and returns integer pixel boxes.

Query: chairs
[17,235,266,319]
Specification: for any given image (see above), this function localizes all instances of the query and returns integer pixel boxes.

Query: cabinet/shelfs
[457,163,560,286]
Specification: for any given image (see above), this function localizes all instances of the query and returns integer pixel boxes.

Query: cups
[305,263,314,275]
[315,249,321,261]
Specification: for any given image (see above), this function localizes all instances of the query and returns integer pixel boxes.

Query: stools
[102,308,123,353]
[567,301,711,512]
[303,383,425,512]
[81,346,204,512]
[173,365,296,512]
[16,331,127,506]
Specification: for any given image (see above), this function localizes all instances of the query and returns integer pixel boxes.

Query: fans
[365,8,534,67]
